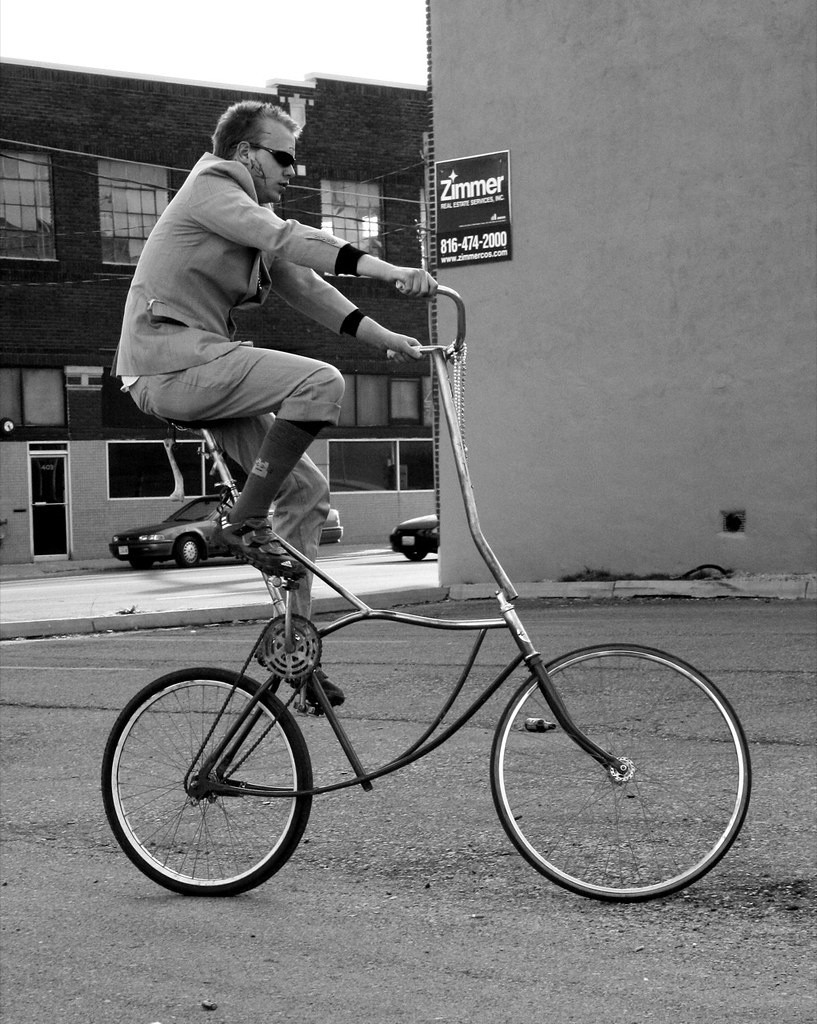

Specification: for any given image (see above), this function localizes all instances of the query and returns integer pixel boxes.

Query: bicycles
[98,281,754,906]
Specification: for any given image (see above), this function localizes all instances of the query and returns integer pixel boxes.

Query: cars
[387,512,440,561]
[107,493,345,568]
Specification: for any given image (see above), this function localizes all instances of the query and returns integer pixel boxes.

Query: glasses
[231,142,296,167]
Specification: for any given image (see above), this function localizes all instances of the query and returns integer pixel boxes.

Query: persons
[109,100,442,704]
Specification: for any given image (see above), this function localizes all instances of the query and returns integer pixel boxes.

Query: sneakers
[257,642,346,707]
[212,512,307,580]
